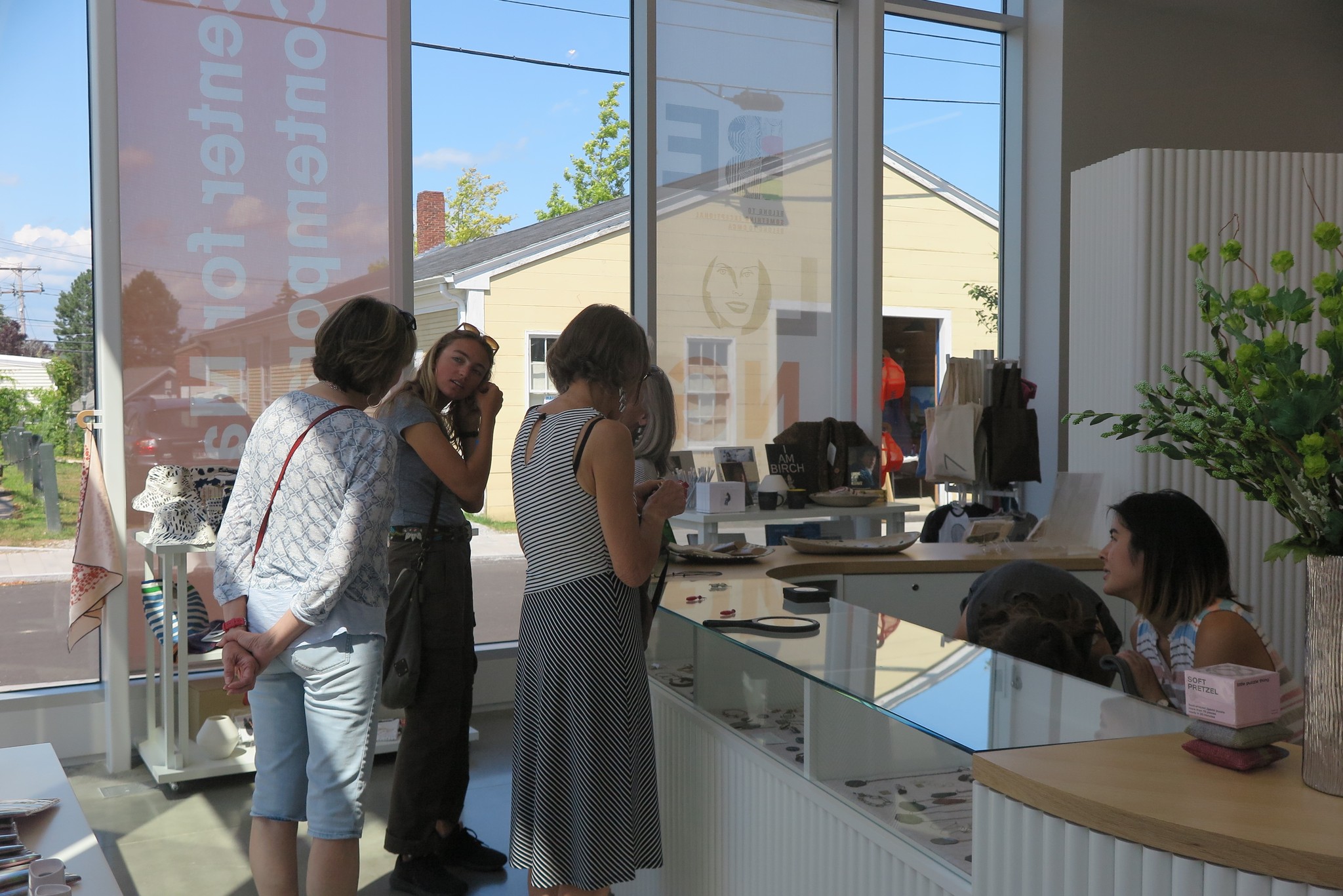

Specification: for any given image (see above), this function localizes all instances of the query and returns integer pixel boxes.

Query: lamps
[903,320,926,333]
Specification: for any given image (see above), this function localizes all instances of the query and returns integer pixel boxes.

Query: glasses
[454,322,499,357]
[390,304,417,330]
[642,364,664,382]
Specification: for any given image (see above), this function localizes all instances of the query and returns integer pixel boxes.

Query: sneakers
[390,853,468,896]
[439,822,506,870]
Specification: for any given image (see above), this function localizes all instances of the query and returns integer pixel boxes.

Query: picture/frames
[235,713,255,743]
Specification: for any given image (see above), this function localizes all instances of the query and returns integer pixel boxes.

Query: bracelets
[222,618,247,634]
[460,430,479,438]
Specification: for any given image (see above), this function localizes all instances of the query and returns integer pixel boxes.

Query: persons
[213,294,419,896]
[1096,488,1305,747]
[375,324,508,896]
[508,306,690,896]
[851,447,877,488]
[617,364,677,521]
[954,559,1123,689]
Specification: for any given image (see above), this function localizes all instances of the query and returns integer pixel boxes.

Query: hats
[879,431,904,487]
[143,500,216,546]
[881,357,906,411]
[132,464,195,513]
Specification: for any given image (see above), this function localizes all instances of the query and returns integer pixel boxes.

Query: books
[718,461,755,509]
[713,446,761,505]
[765,443,806,490]
[847,446,881,489]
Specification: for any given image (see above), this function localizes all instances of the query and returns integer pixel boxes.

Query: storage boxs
[1184,662,1281,729]
[696,481,745,513]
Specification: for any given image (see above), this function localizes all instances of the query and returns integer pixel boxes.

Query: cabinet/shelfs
[133,528,403,790]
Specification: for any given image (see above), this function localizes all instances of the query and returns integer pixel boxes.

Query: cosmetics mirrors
[703,615,821,633]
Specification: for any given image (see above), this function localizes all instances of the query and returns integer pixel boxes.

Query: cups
[27,859,71,896]
[758,491,783,510]
[788,489,811,509]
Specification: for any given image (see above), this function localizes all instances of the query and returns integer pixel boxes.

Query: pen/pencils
[671,466,715,508]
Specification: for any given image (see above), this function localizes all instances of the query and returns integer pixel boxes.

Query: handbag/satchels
[915,355,1042,485]
[378,551,428,710]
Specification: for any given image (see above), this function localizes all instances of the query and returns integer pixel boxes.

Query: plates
[668,542,773,564]
[782,532,921,553]
[810,492,879,508]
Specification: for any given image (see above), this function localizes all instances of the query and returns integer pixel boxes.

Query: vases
[1301,553,1343,798]
[196,715,239,761]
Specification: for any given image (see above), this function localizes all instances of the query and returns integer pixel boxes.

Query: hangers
[949,483,1027,519]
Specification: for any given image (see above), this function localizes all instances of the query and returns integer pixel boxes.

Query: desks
[668,501,920,544]
[0,743,124,896]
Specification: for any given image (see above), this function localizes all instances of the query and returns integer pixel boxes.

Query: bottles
[196,715,241,760]
[759,474,789,507]
